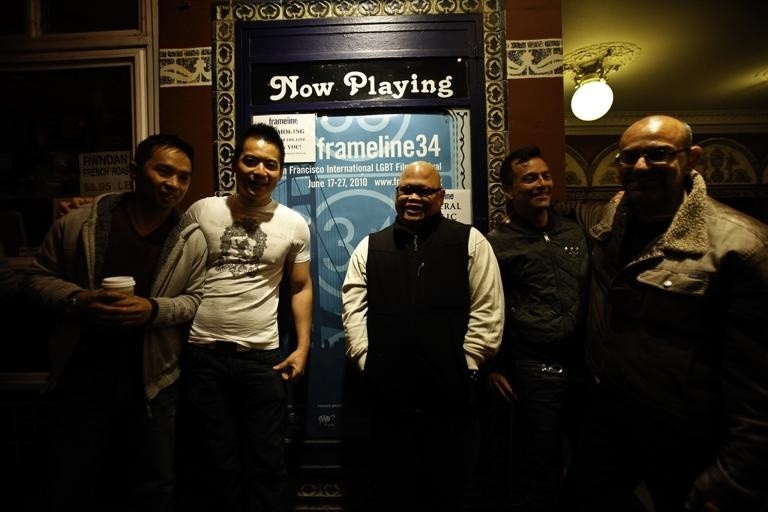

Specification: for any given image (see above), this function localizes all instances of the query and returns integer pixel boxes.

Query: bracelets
[70,295,79,309]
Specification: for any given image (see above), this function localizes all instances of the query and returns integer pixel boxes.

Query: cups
[101,276,136,296]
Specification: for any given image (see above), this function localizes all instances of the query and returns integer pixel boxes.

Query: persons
[47,124,314,512]
[24,132,209,510]
[342,162,509,512]
[582,108,767,512]
[475,145,606,512]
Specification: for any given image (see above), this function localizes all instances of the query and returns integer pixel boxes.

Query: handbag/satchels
[516,358,583,432]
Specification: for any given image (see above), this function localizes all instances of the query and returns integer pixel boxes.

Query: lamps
[563,41,642,121]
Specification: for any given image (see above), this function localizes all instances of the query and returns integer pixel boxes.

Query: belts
[194,341,252,352]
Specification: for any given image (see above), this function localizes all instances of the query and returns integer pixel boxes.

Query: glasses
[621,146,688,164]
[396,185,443,197]
